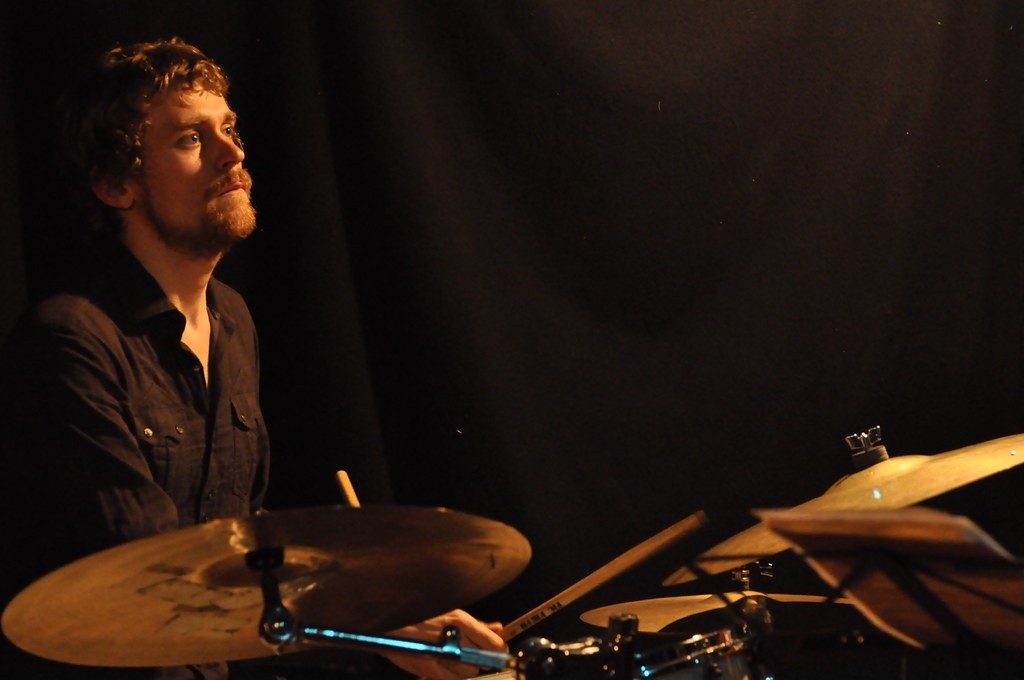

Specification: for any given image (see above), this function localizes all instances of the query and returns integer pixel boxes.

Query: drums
[518,622,787,679]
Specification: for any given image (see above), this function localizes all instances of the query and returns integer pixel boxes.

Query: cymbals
[1,497,534,669]
[580,589,853,635]
[662,431,1024,588]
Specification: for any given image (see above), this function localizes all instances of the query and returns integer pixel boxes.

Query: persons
[0,35,509,680]
